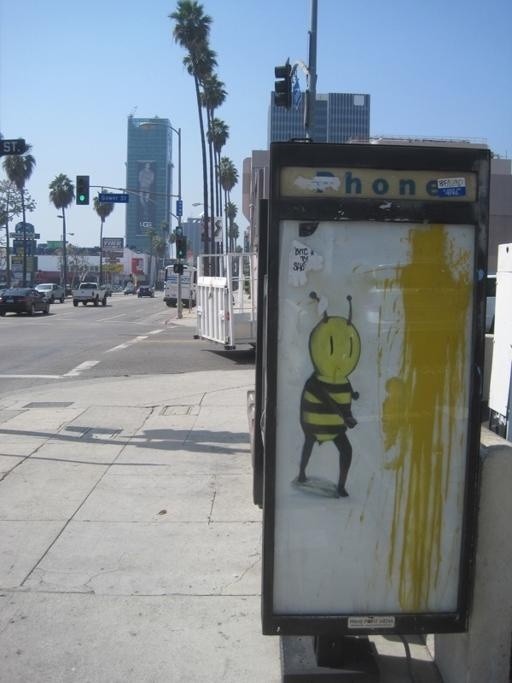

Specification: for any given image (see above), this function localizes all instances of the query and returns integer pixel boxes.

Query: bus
[162,265,198,309]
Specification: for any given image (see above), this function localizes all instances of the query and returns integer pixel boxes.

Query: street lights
[191,201,211,209]
[55,214,67,282]
[59,232,77,281]
[138,119,184,320]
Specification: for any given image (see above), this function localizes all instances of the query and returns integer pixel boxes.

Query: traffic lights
[175,234,188,259]
[272,55,294,113]
[75,175,90,205]
[173,263,184,274]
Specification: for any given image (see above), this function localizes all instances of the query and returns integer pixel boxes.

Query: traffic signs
[0,138,26,157]
[98,193,129,204]
[46,240,65,249]
[176,199,183,217]
[291,82,303,112]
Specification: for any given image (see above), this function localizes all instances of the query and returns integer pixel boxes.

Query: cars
[484,268,497,335]
[0,280,165,319]
